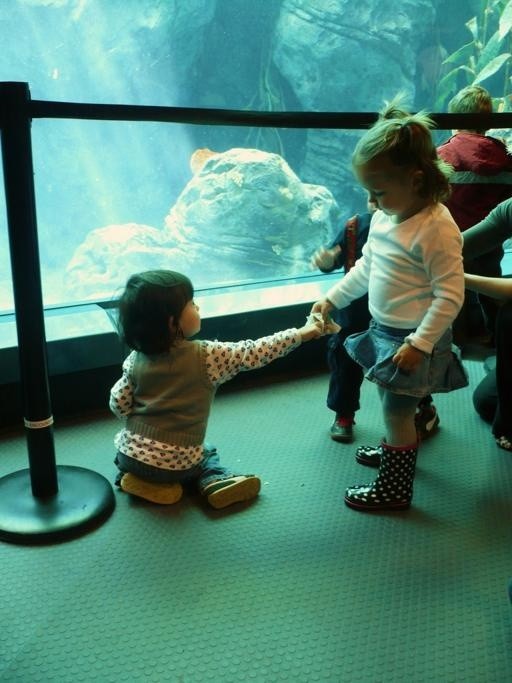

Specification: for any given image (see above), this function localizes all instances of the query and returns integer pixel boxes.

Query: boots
[344,431,421,512]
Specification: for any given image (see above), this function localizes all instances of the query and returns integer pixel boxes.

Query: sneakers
[120,472,183,505]
[330,412,355,441]
[415,406,439,439]
[204,475,260,509]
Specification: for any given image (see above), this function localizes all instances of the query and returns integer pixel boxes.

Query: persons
[310,194,439,444]
[108,269,330,504]
[434,84,511,361]
[311,87,469,512]
[461,198,511,451]
